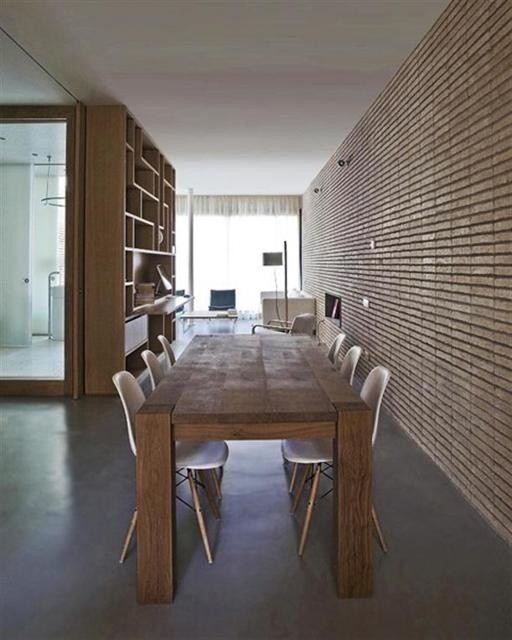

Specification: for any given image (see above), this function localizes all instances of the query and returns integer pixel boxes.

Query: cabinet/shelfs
[85,107,194,394]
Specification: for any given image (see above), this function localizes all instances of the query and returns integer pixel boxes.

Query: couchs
[260,289,315,328]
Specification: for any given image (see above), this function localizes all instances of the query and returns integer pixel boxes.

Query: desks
[134,335,373,604]
[180,311,238,334]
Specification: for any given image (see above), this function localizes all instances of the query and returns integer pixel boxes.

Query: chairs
[208,289,236,320]
[281,366,391,558]
[112,370,229,563]
[329,332,346,364]
[252,313,315,337]
[141,350,164,391]
[341,346,362,386]
[157,334,176,368]
